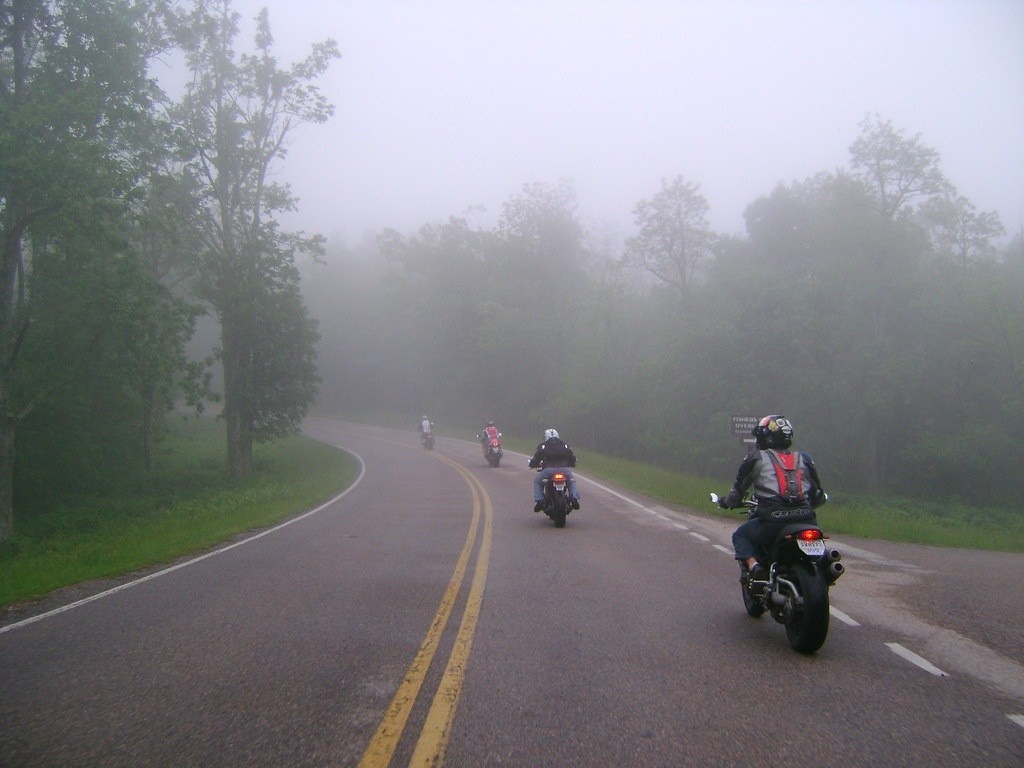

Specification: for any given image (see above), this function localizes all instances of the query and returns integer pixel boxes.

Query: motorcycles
[417,422,436,450]
[709,489,842,656]
[476,433,503,469]
[527,457,579,527]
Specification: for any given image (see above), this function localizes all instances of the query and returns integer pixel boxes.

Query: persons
[480,419,503,459]
[528,428,581,513]
[718,414,827,596]
[419,415,433,443]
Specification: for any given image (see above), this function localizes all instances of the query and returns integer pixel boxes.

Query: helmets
[421,416,427,421]
[544,429,559,441]
[487,419,494,426]
[751,415,793,451]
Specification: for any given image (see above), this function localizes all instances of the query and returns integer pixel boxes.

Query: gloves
[719,497,730,509]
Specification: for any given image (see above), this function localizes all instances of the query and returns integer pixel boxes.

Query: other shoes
[534,500,544,512]
[572,497,580,510]
[747,563,764,599]
